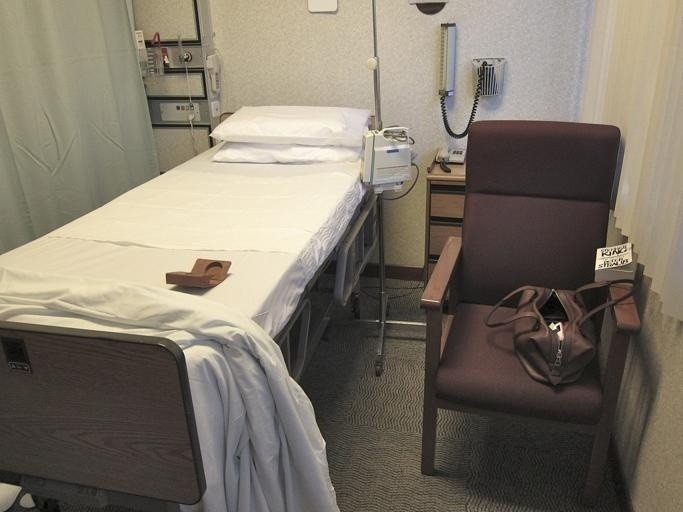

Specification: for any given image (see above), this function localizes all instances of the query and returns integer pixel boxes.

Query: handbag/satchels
[484,279,639,388]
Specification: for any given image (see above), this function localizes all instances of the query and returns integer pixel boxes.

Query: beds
[0,104,381,510]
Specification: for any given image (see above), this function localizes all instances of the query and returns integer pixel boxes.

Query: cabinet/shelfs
[425,151,465,288]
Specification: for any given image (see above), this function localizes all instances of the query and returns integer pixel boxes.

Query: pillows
[212,143,364,165]
[208,104,371,147]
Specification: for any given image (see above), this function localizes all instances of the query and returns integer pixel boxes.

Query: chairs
[414,119,645,497]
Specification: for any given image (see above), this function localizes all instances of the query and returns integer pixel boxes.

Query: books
[594,242,638,283]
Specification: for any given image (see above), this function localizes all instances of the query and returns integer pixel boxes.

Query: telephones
[436,145,466,163]
